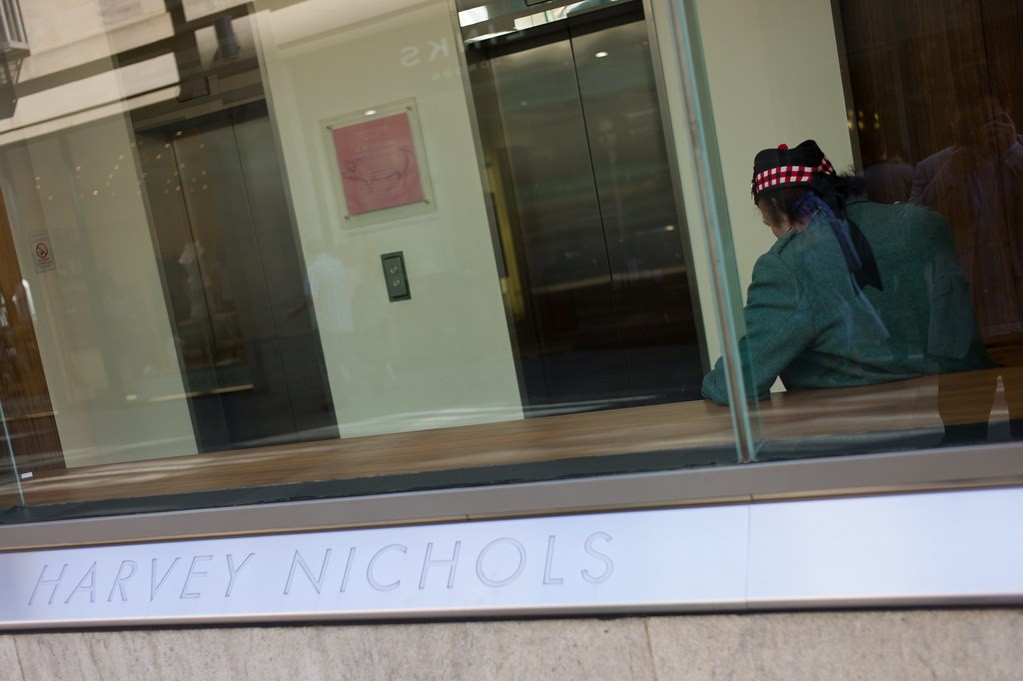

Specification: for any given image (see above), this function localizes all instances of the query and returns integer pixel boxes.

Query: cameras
[959,115,992,148]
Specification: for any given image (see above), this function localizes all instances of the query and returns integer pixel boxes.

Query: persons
[588,112,642,237]
[0,334,51,416]
[859,62,1023,446]
[699,139,1000,407]
[164,267,249,378]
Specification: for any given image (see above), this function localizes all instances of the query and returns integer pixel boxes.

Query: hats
[750,139,837,205]
